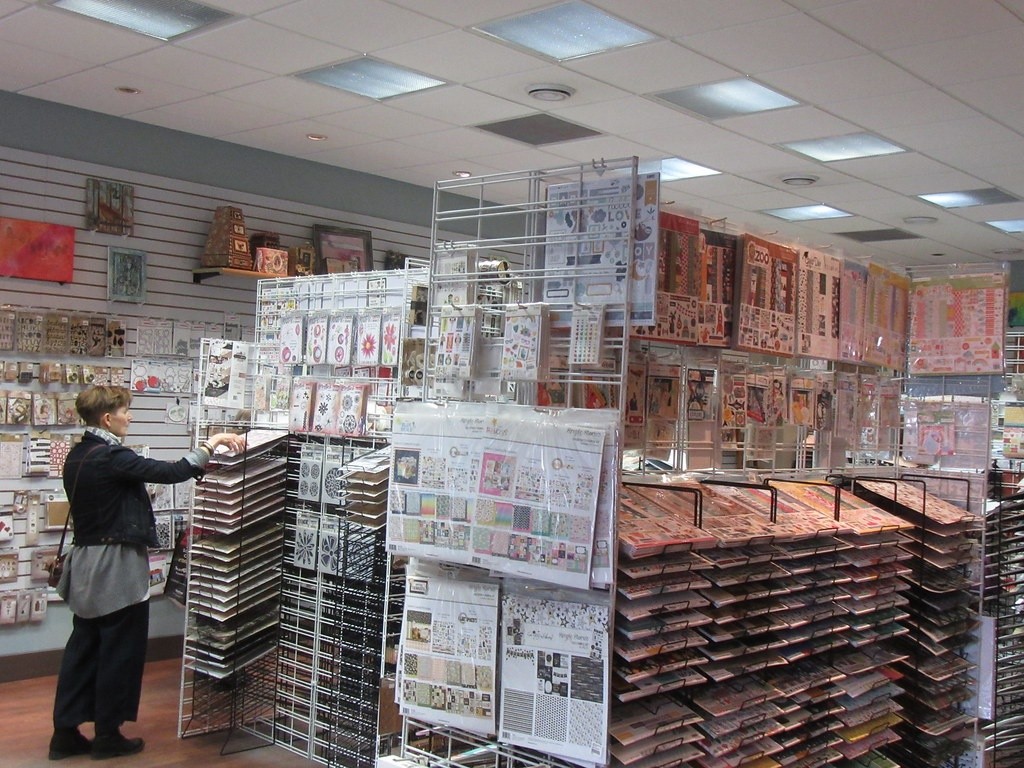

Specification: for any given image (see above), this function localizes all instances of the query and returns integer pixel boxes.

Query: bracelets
[200,440,215,457]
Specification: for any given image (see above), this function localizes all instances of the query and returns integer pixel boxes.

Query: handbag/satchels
[47,552,69,588]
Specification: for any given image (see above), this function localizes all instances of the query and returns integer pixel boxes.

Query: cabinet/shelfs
[175,151,1024,768]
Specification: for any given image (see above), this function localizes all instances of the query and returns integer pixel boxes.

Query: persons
[48,385,250,760]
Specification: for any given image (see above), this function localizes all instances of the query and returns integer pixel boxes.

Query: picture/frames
[107,246,148,305]
[313,223,373,276]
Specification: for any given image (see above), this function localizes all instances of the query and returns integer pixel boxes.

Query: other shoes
[48,730,91,760]
[91,732,145,760]
